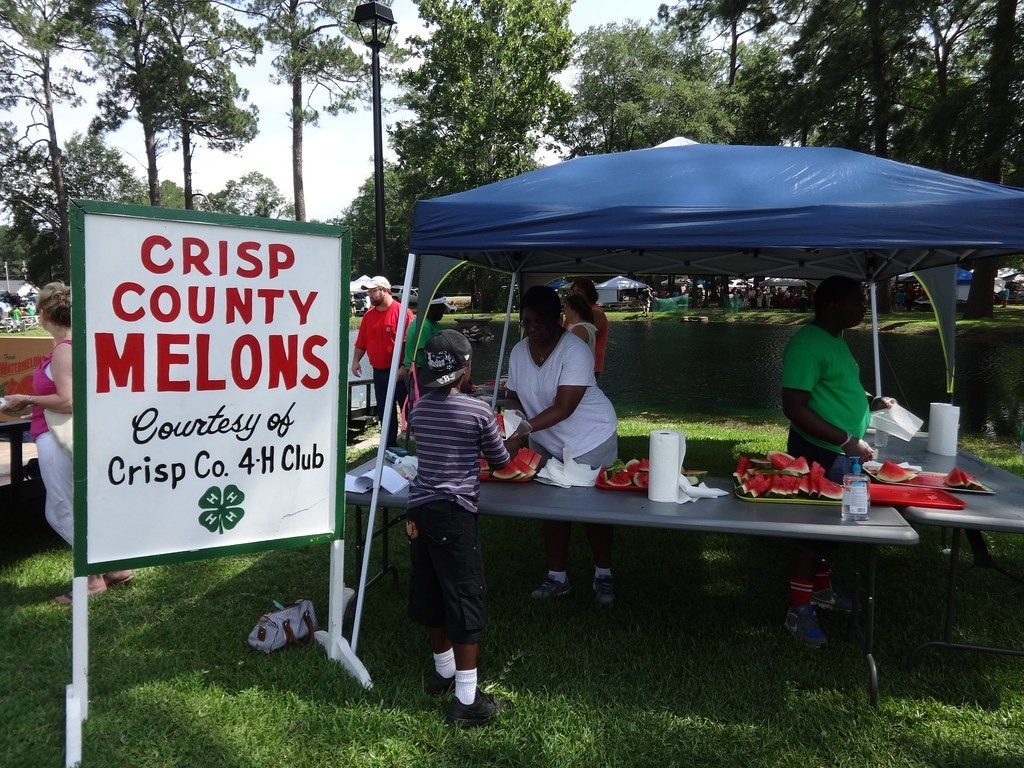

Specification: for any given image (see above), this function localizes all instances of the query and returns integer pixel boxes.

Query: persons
[564,294,598,357]
[890,282,927,311]
[1000,284,1019,308]
[781,276,897,648]
[8,293,35,334]
[0,283,134,605]
[352,276,414,447]
[402,294,455,391]
[504,285,618,609]
[405,329,514,727]
[730,286,816,313]
[620,281,703,317]
[362,292,371,312]
[562,279,608,380]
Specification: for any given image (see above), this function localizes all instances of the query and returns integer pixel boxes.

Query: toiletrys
[840,454,872,521]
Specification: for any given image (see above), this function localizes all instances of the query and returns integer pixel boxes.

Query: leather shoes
[447,687,513,725]
[428,671,456,694]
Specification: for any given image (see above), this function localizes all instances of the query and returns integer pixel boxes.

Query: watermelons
[495,413,506,439]
[944,466,983,491]
[600,457,707,488]
[732,450,844,501]
[480,447,543,480]
[875,460,920,482]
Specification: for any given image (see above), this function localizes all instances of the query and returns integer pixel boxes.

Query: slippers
[104,573,132,587]
[53,591,72,602]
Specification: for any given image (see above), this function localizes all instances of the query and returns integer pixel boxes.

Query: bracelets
[402,363,408,372]
[29,396,33,406]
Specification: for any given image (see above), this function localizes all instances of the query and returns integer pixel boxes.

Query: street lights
[350,2,396,285]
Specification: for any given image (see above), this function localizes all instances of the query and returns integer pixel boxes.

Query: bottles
[875,427,888,447]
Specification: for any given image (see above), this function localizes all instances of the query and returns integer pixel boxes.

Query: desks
[479,374,509,414]
[858,433,1024,676]
[0,417,31,487]
[346,474,919,713]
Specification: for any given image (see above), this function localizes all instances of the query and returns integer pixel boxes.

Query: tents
[594,275,647,301]
[350,275,372,293]
[661,276,805,293]
[352,143,1024,658]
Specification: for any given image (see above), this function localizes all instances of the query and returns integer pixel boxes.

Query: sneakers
[593,574,615,604]
[785,605,829,648]
[810,580,863,612]
[532,576,570,597]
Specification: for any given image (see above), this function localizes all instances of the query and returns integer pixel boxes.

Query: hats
[420,329,472,387]
[361,276,391,290]
[430,296,452,309]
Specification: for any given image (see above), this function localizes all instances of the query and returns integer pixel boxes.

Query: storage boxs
[0,335,57,423]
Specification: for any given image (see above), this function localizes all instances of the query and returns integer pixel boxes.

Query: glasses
[571,288,585,293]
[520,317,546,327]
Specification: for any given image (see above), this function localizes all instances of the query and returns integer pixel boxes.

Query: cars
[390,286,422,304]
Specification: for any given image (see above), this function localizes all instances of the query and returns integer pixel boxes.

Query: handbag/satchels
[249,599,317,653]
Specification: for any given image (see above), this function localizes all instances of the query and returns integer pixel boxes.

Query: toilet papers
[927,402,960,458]
[648,429,686,501]
